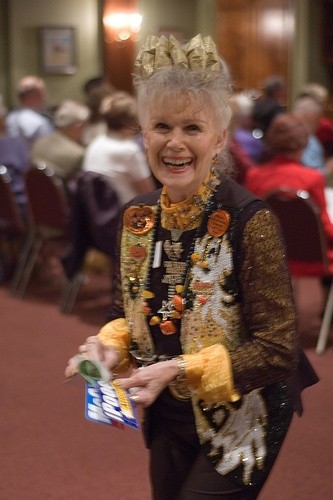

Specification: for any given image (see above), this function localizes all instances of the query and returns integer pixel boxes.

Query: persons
[0,76,333,318]
[61,32,319,500]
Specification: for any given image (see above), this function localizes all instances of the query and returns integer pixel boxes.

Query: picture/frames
[34,23,79,77]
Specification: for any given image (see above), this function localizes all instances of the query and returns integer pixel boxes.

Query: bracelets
[174,355,186,384]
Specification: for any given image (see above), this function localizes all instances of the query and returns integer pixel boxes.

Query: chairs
[266,189,333,353]
[0,163,123,313]
